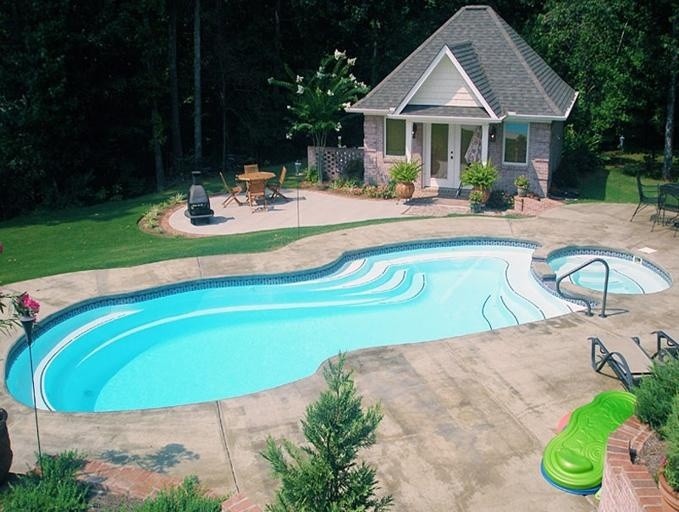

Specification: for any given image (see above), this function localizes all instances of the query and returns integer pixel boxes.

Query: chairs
[220,163,290,215]
[585,323,679,396]
[629,170,679,234]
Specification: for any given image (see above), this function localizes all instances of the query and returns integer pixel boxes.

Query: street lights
[18,310,43,478]
[293,158,302,240]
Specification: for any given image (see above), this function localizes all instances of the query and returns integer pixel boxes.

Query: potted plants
[388,154,424,200]
[513,174,531,198]
[459,156,501,214]
[656,389,679,512]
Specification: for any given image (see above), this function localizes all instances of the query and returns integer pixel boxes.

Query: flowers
[0,290,42,338]
